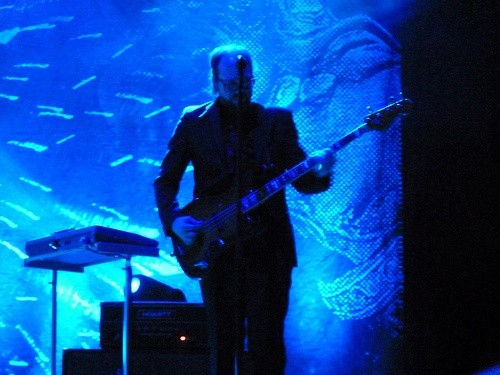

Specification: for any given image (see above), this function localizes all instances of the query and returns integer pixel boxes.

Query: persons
[154,46,335,375]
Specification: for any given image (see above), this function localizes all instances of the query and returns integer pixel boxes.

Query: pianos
[22,223,160,273]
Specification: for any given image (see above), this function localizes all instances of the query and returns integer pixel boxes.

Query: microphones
[234,55,249,72]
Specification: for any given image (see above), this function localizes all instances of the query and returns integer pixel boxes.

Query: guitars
[170,93,413,279]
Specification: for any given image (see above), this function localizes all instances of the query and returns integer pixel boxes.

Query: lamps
[123,274,187,301]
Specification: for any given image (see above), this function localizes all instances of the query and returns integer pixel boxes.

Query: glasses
[217,78,254,93]
